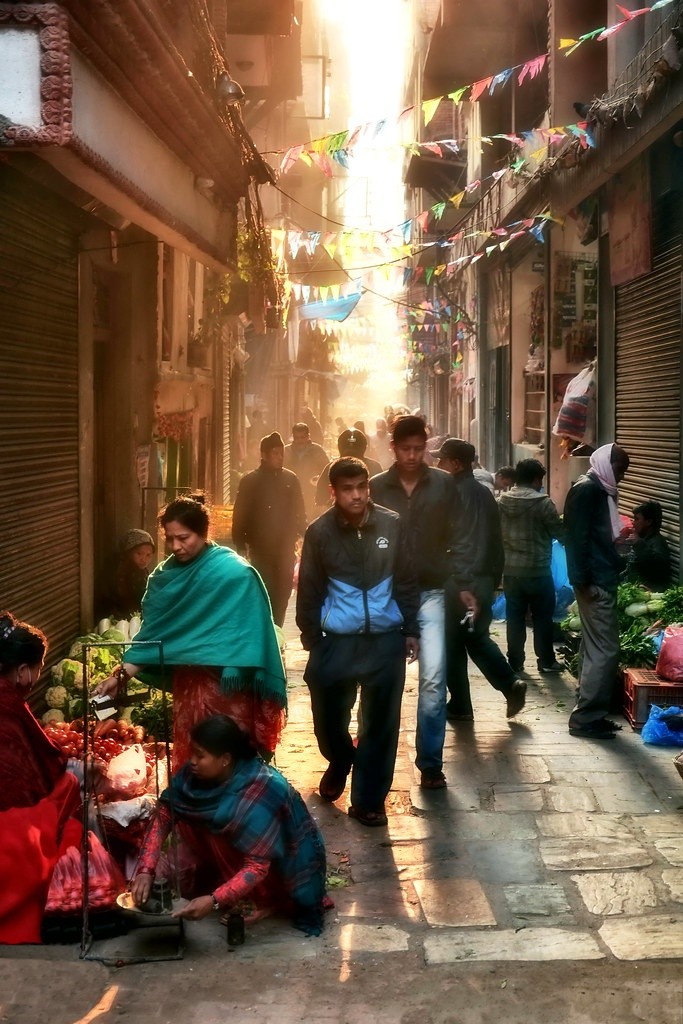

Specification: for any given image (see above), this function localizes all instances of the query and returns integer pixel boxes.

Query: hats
[429,437,475,462]
[293,423,308,436]
[118,529,156,556]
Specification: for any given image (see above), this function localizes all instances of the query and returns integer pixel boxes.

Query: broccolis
[43,642,97,725]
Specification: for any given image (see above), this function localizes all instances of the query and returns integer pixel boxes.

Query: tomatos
[45,728,158,775]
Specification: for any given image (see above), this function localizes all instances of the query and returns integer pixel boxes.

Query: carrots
[44,719,158,744]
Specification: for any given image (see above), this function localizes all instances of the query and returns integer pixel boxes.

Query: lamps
[217,71,245,106]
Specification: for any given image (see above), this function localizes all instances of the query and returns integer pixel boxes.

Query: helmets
[338,429,367,457]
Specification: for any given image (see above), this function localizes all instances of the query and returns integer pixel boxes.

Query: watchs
[209,894,220,911]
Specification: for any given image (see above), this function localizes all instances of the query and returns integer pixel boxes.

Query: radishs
[98,617,141,641]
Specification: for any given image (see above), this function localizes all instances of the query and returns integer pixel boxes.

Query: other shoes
[568,721,616,739]
[421,771,447,790]
[604,718,623,730]
[348,805,388,826]
[539,661,567,673]
[220,901,273,926]
[319,745,357,801]
[446,703,473,721]
[323,893,334,911]
[506,679,527,717]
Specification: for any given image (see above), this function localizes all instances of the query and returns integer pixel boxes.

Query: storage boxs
[623,667,683,732]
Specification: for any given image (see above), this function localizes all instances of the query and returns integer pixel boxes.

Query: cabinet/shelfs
[523,371,546,431]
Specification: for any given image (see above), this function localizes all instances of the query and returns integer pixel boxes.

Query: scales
[79,639,191,965]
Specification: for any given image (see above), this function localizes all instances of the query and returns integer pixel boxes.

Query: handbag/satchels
[44,829,128,914]
[655,625,683,682]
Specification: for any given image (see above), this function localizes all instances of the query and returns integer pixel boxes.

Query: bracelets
[136,866,155,880]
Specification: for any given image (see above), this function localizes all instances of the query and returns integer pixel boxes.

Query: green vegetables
[558,582,683,677]
[130,689,176,745]
[72,630,147,691]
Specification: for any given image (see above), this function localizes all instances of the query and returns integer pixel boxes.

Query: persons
[98,497,290,779]
[296,455,421,827]
[0,612,126,941]
[242,404,669,739]
[365,414,481,788]
[133,712,336,927]
